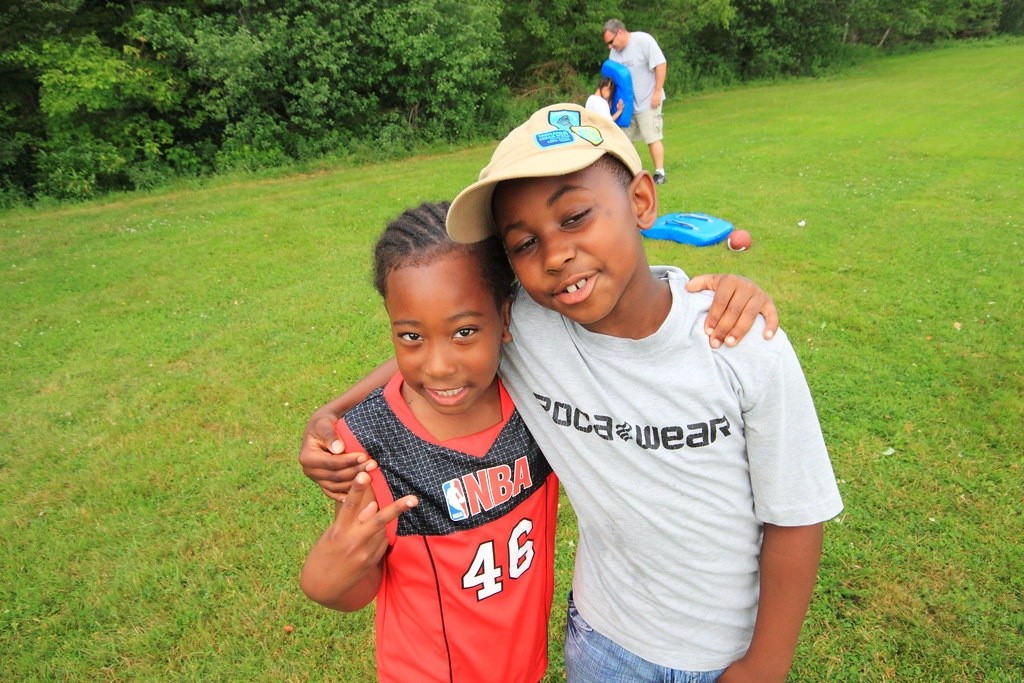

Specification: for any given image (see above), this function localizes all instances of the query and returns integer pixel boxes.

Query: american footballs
[726,230,752,251]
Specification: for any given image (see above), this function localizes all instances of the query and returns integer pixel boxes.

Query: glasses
[606,34,617,45]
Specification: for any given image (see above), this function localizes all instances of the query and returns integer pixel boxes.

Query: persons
[603,19,668,185]
[584,78,625,122]
[302,201,779,683]
[297,102,844,683]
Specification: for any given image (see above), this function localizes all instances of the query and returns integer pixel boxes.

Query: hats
[444,101,644,245]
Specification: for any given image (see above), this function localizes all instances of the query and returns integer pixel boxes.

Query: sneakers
[652,170,666,185]
